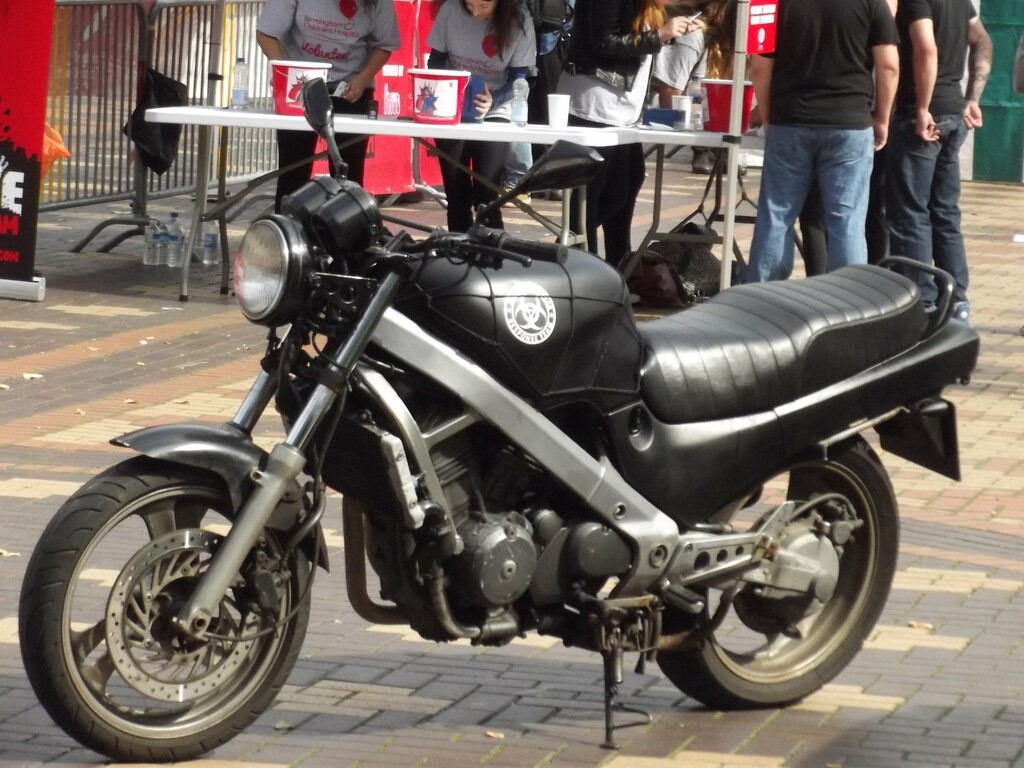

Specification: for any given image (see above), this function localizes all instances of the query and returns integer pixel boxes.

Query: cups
[672,95,694,127]
[547,93,571,127]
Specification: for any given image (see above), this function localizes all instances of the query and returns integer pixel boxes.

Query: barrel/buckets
[701,79,754,133]
[408,68,471,125]
[270,59,332,116]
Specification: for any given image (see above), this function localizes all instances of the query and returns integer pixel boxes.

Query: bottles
[367,100,378,120]
[203,220,219,265]
[511,72,529,126]
[143,212,186,268]
[232,58,249,110]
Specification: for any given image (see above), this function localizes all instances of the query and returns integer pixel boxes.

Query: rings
[345,87,350,91]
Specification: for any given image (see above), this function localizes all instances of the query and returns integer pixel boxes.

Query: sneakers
[952,300,970,327]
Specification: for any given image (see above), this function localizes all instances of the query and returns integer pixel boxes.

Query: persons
[692,146,745,174]
[256,0,402,215]
[425,0,737,268]
[747,0,993,325]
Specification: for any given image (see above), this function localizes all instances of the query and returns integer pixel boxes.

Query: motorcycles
[18,74,982,764]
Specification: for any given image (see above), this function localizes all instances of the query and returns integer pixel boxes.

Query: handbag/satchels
[526,2,575,57]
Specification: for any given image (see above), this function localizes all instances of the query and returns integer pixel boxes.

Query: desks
[621,128,765,283]
[146,107,637,301]
